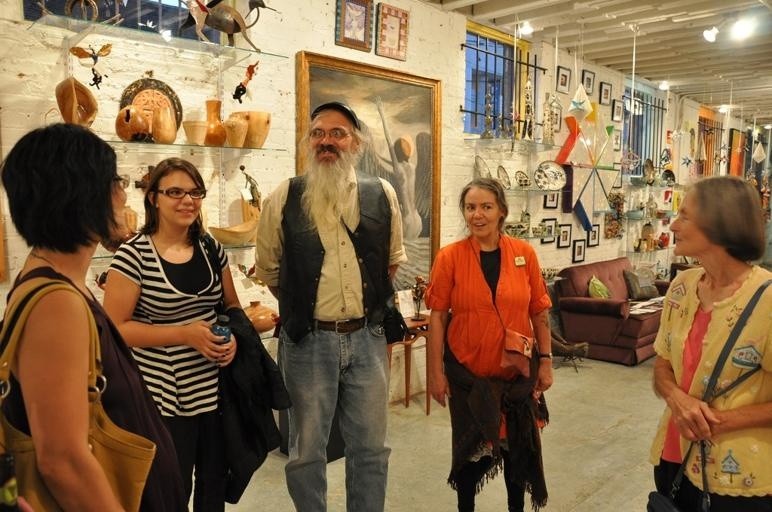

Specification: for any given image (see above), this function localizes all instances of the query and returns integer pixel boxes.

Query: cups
[182,119,209,147]
[504,223,529,238]
[531,226,543,237]
[151,106,177,144]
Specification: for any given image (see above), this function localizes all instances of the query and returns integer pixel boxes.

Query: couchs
[552,251,675,369]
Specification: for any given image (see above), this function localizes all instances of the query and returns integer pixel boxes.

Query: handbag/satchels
[501,329,534,377]
[385,308,408,342]
[647,493,680,511]
[1,276,157,511]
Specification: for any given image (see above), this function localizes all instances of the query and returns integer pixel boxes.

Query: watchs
[539,352,553,359]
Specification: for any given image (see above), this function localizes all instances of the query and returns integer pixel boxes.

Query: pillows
[587,272,612,302]
[620,264,661,301]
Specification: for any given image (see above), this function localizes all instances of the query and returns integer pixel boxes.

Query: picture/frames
[374,3,412,64]
[291,49,443,305]
[333,1,377,55]
[535,186,601,264]
[546,64,626,190]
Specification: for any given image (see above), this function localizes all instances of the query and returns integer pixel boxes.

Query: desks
[382,310,439,419]
[669,262,699,283]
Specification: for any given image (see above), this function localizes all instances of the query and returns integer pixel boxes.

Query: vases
[240,299,281,335]
[175,93,278,152]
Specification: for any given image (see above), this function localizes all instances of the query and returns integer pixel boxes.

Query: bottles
[659,232,670,246]
[225,111,250,148]
[540,268,559,278]
[203,98,225,147]
[641,222,655,251]
[241,301,278,334]
[645,193,658,217]
[211,314,233,349]
[539,222,548,237]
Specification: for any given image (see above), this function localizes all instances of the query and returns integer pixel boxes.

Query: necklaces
[29,252,56,267]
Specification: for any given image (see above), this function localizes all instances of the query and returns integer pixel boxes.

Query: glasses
[310,130,347,138]
[110,174,131,190]
[154,187,206,200]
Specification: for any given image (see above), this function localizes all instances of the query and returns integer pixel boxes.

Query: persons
[254,102,407,512]
[102,157,242,512]
[424,178,553,512]
[0,123,187,512]
[649,175,772,512]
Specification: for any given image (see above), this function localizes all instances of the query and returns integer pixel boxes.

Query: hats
[311,102,360,130]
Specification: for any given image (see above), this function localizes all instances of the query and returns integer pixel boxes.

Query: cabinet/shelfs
[461,132,565,244]
[31,10,290,343]
[619,182,679,255]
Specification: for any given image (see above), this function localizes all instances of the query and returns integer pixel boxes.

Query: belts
[312,316,369,332]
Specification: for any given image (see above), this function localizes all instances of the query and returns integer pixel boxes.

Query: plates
[119,77,182,131]
[496,165,511,189]
[643,158,675,186]
[473,155,492,180]
[534,169,550,191]
[539,160,568,191]
[514,170,529,186]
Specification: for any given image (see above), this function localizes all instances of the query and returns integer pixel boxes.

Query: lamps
[700,17,734,45]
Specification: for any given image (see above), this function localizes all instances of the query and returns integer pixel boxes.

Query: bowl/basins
[229,110,273,148]
[626,210,644,219]
[631,176,646,185]
